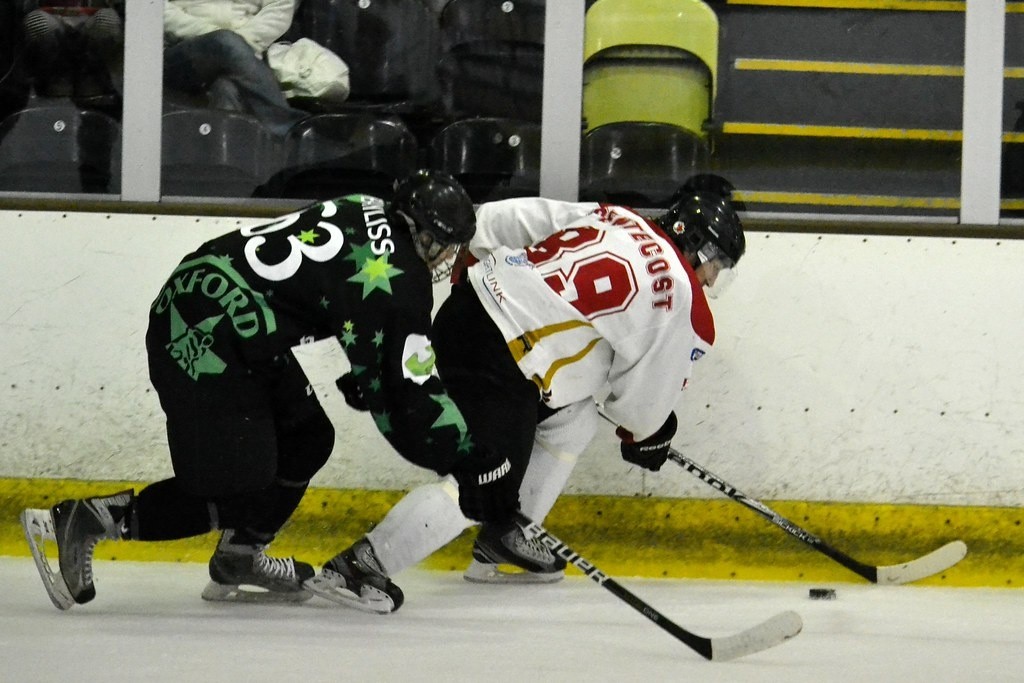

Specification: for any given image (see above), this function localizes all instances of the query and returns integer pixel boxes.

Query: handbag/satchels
[267,37,350,106]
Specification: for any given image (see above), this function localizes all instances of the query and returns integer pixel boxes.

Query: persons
[162,0,315,132]
[15,0,125,99]
[300,172,748,611]
[24,169,524,611]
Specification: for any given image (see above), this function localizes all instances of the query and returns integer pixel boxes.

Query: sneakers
[462,518,566,584]
[201,529,316,603]
[302,536,404,614]
[20,488,135,610]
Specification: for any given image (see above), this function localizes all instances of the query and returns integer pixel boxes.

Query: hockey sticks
[450,465,805,664]
[594,397,969,586]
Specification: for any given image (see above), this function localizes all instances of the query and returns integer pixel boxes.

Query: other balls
[808,587,835,601]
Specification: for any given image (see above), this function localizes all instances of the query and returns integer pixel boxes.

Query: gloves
[452,438,521,524]
[615,409,678,471]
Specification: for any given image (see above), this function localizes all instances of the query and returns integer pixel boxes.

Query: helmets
[390,168,476,284]
[654,190,745,270]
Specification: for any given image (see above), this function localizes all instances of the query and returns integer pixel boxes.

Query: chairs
[0,106,120,194]
[438,0,546,125]
[582,121,710,208]
[110,111,280,199]
[290,0,438,119]
[280,113,421,200]
[429,118,542,203]
[581,1,718,155]
